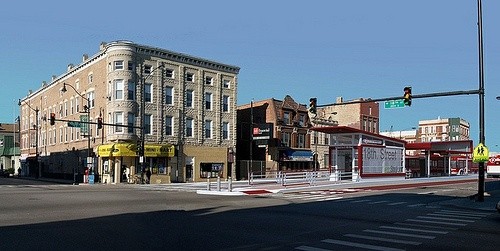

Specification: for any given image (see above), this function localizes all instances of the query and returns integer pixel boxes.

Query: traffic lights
[50,112,56,125]
[309,98,317,113]
[97,117,103,129]
[403,85,412,106]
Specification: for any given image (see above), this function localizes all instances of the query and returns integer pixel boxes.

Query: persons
[122,166,151,184]
[18,167,22,176]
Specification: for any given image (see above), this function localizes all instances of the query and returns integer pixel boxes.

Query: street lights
[61,82,91,157]
[128,64,165,184]
[17,98,40,179]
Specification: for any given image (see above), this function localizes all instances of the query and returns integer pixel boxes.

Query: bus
[449,155,487,176]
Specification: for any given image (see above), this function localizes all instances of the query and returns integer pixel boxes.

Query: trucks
[486,154,500,178]
